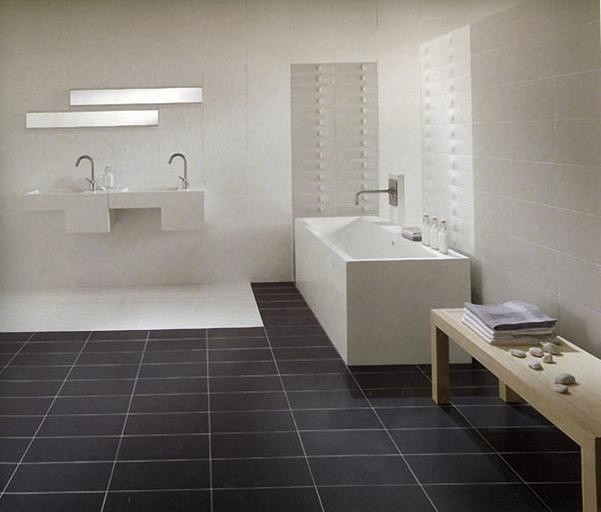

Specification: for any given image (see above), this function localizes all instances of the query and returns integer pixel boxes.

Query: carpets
[0,282,264,334]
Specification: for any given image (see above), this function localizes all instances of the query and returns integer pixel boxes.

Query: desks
[429,304,601,512]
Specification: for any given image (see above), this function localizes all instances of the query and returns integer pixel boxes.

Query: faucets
[168,153,188,188]
[75,154,96,191]
[355,189,394,205]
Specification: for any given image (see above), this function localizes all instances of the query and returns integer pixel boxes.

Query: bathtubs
[294,214,472,366]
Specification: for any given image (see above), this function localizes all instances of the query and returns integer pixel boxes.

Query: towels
[458,299,556,350]
